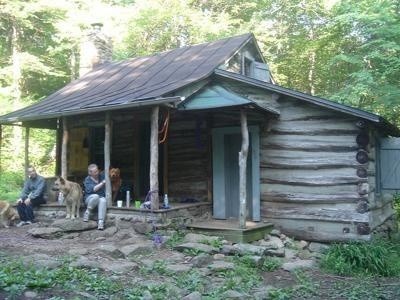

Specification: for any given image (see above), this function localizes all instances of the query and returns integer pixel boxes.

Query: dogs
[51,175,83,220]
[110,167,122,202]
[0,200,20,228]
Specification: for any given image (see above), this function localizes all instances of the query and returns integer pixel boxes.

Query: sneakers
[16,221,25,227]
[97,220,105,230]
[83,209,90,222]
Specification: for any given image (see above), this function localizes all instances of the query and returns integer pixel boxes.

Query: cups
[135,201,141,208]
[117,200,123,208]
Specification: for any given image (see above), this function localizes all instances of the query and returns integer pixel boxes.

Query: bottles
[58,192,64,205]
[164,194,169,206]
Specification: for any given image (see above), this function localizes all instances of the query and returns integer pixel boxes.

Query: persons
[82,163,108,230]
[16,166,47,227]
